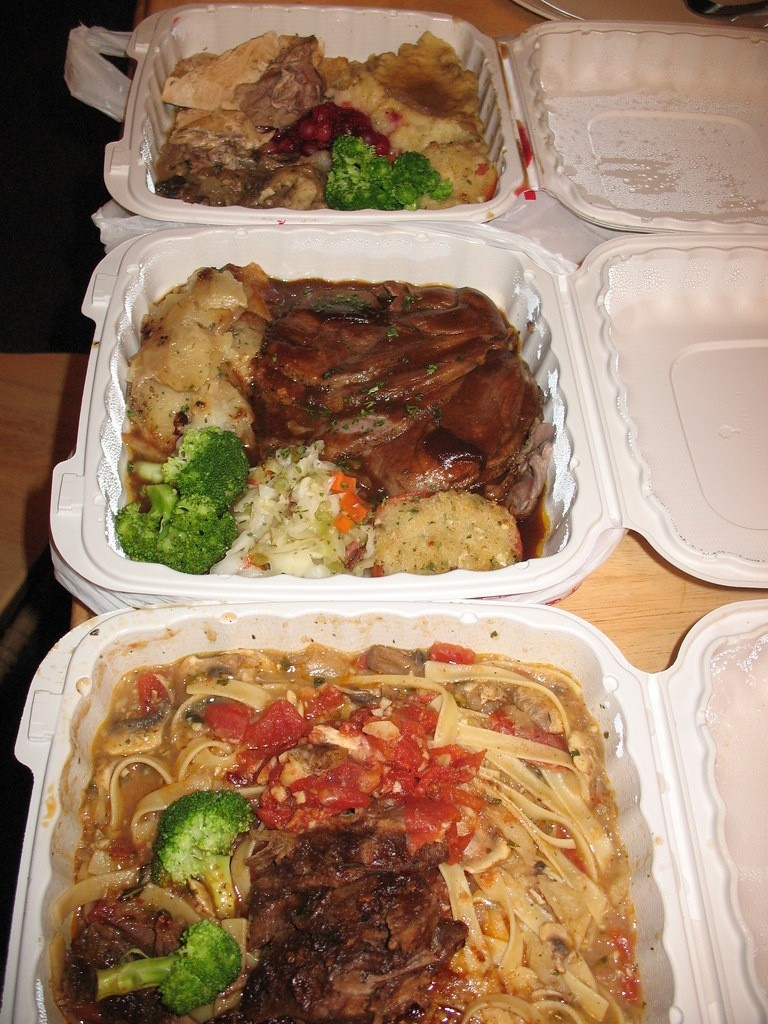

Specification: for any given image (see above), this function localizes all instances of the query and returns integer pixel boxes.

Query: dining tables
[132,0,768,84]
[0,351,767,674]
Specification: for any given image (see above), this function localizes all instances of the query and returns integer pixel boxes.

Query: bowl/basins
[49,220,767,611]
[105,2,765,233]
[3,596,768,1024]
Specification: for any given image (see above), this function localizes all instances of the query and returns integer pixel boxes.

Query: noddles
[39,655,643,1024]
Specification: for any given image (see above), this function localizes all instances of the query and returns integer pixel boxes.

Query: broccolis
[116,426,234,576]
[326,135,451,210]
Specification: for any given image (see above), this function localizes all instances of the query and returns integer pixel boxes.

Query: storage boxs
[45,217,767,616]
[97,2,767,239]
[2,598,767,1022]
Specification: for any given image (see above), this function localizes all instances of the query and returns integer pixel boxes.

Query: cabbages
[214,444,364,576]
